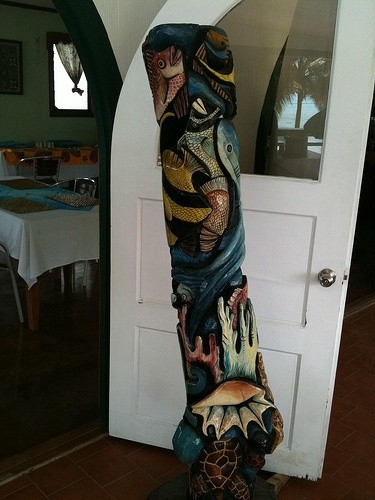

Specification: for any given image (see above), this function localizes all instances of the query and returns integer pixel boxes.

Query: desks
[0,145,99,179]
[0,176,99,331]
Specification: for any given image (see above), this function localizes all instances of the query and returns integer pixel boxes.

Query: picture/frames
[0,39,25,94]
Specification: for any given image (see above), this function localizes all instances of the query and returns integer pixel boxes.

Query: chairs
[0,155,98,323]
